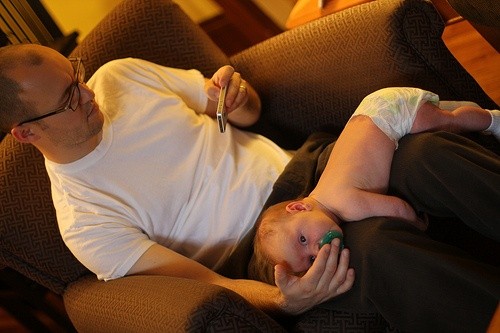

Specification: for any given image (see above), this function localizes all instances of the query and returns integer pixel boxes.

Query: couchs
[0,0,500,333]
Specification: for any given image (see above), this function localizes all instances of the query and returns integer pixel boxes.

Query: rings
[240,85,248,92]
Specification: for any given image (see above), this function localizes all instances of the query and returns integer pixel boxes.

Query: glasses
[16,57,85,128]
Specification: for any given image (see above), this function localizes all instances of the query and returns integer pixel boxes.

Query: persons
[254,87,500,285]
[0,43,500,333]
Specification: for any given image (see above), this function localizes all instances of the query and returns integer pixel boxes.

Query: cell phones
[217,83,228,134]
